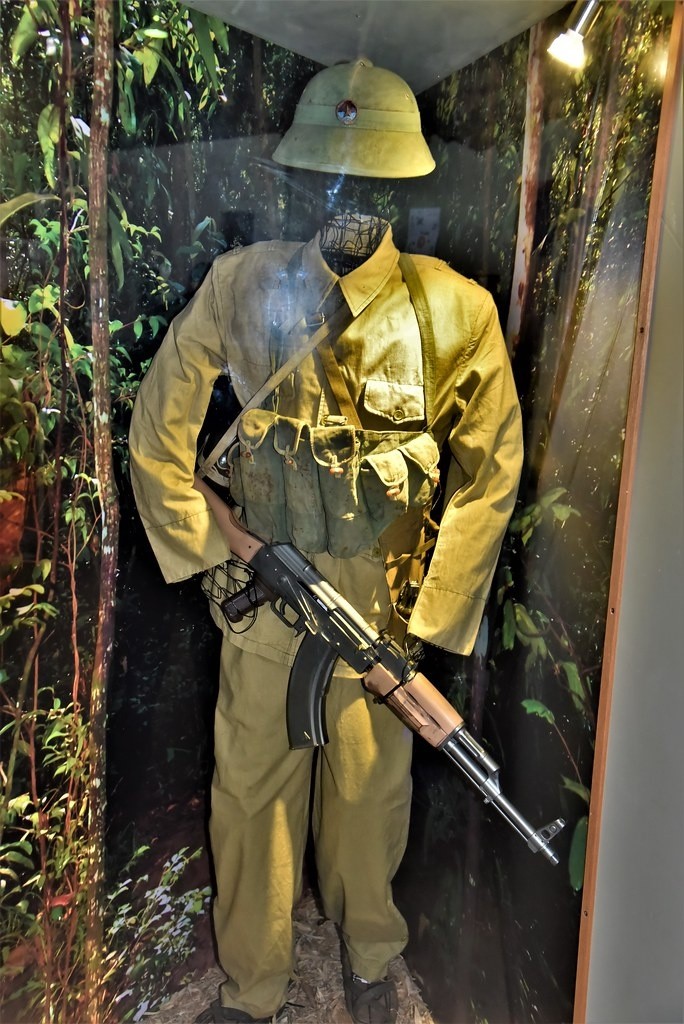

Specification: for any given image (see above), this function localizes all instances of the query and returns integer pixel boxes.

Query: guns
[190,472,566,871]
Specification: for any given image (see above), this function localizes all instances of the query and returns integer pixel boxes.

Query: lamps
[548,0,603,73]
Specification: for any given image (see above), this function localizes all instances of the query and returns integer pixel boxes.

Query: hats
[271,59,436,177]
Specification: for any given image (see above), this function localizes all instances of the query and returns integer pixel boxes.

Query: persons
[117,51,530,1024]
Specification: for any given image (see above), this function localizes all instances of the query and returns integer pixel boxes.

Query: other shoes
[341,945,399,1024]
[203,1004,285,1024]
